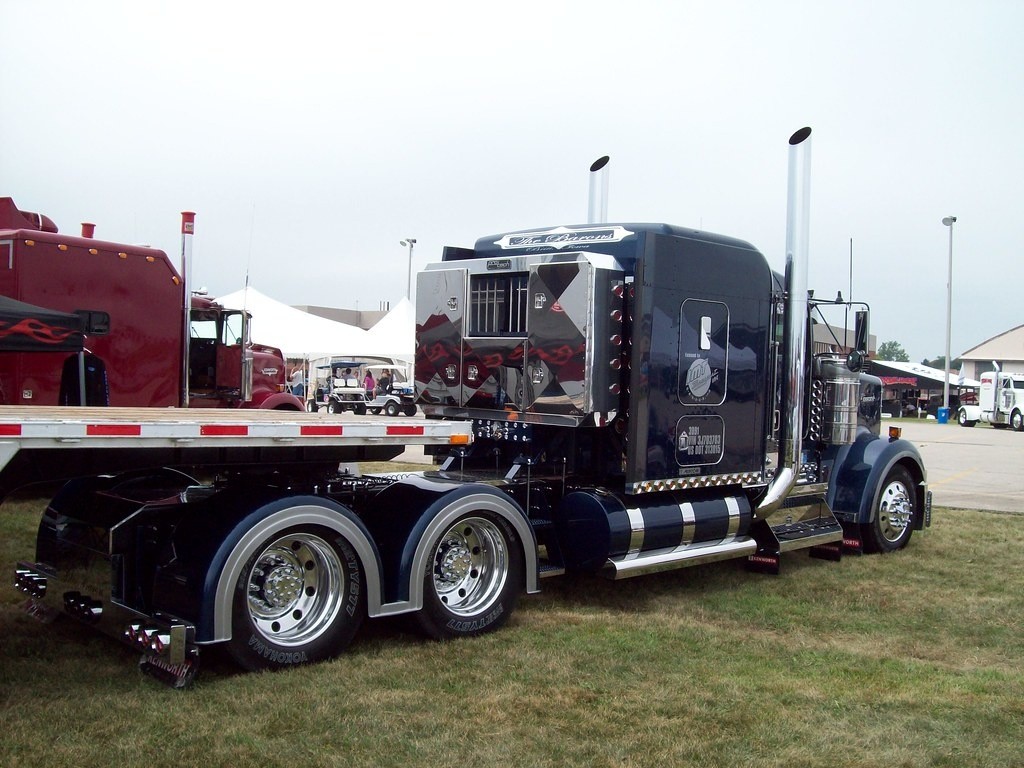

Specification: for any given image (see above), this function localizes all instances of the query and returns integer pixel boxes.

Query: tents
[188,287,416,404]
[864,360,980,419]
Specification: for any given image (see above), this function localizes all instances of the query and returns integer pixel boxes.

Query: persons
[326,367,389,400]
[290,366,303,395]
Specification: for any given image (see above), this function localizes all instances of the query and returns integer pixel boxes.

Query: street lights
[937,215,959,423]
[397,236,416,301]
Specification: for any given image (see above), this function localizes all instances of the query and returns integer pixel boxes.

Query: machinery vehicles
[958,361,1024,432]
[0,192,309,484]
[8,123,933,688]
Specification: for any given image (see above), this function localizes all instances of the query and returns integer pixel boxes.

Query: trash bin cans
[938,406,951,424]
[316,388,329,402]
